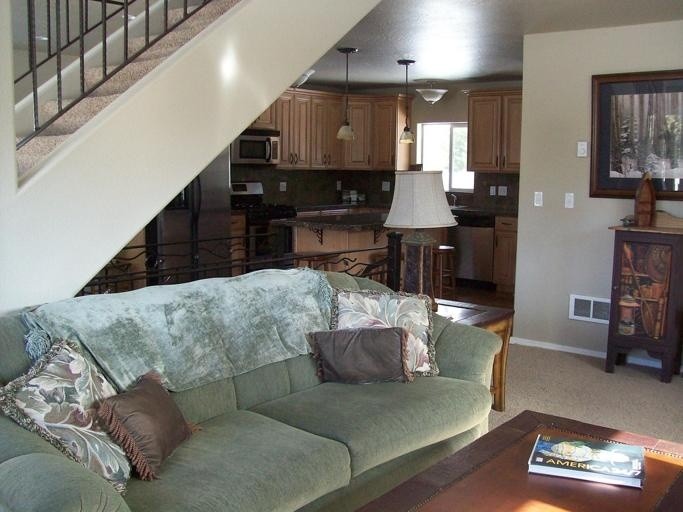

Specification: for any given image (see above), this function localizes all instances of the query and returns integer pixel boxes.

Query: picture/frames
[587,70,683,201]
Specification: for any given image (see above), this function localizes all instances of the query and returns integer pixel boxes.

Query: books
[528,434,644,488]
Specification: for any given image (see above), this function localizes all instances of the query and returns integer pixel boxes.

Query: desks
[406,299,516,413]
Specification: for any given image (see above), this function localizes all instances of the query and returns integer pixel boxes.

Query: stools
[427,245,462,302]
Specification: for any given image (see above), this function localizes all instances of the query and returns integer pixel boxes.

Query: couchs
[1,268,503,512]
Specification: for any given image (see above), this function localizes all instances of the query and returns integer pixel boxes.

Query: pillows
[99,372,198,478]
[0,336,134,494]
[307,327,412,385]
[326,290,441,378]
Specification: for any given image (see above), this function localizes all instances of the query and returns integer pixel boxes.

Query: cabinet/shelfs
[339,97,374,169]
[227,213,250,279]
[373,92,412,171]
[464,91,522,172]
[275,90,312,169]
[491,214,519,296]
[310,90,346,168]
[595,221,681,380]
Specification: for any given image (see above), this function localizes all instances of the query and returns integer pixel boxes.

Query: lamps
[293,68,316,88]
[417,80,449,105]
[380,170,459,313]
[396,59,415,143]
[336,47,361,141]
